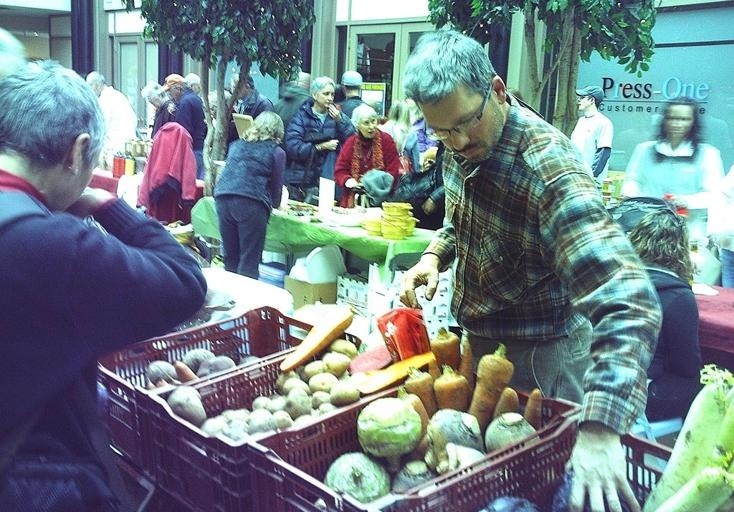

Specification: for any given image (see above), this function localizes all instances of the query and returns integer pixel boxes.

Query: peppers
[147,338,360,442]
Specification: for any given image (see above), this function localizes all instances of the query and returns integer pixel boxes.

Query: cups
[422,83,490,143]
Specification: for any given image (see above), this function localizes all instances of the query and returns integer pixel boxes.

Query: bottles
[159,73,185,91]
[576,86,605,101]
[340,71,363,86]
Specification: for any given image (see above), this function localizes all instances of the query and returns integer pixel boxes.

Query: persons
[85,72,136,170]
[571,86,613,200]
[337,71,363,119]
[224,72,275,156]
[622,200,703,422]
[164,74,208,179]
[621,97,724,237]
[334,102,402,208]
[0,59,209,512]
[185,74,201,95]
[707,163,734,289]
[282,76,354,205]
[209,89,233,129]
[141,83,174,138]
[400,28,662,512]
[378,96,447,231]
[274,72,311,122]
[213,111,286,278]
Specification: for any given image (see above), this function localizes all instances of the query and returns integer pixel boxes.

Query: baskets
[95,306,673,511]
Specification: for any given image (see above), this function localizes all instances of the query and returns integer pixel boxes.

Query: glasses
[422,83,490,143]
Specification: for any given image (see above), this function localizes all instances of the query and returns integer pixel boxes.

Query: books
[284,275,337,311]
[335,265,452,336]
[255,258,285,288]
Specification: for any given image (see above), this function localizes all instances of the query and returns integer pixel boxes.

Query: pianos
[278,305,358,373]
[396,326,543,463]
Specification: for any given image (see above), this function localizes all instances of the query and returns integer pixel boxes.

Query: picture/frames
[690,275,732,375]
[87,165,119,193]
[186,191,440,270]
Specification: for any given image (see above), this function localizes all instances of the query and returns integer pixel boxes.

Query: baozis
[551,472,637,512]
[489,495,539,512]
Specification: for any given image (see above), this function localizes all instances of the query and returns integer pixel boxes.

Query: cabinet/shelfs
[111,150,135,177]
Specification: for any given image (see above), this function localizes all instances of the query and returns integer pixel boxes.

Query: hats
[159,73,185,91]
[576,86,605,101]
[340,71,363,86]
